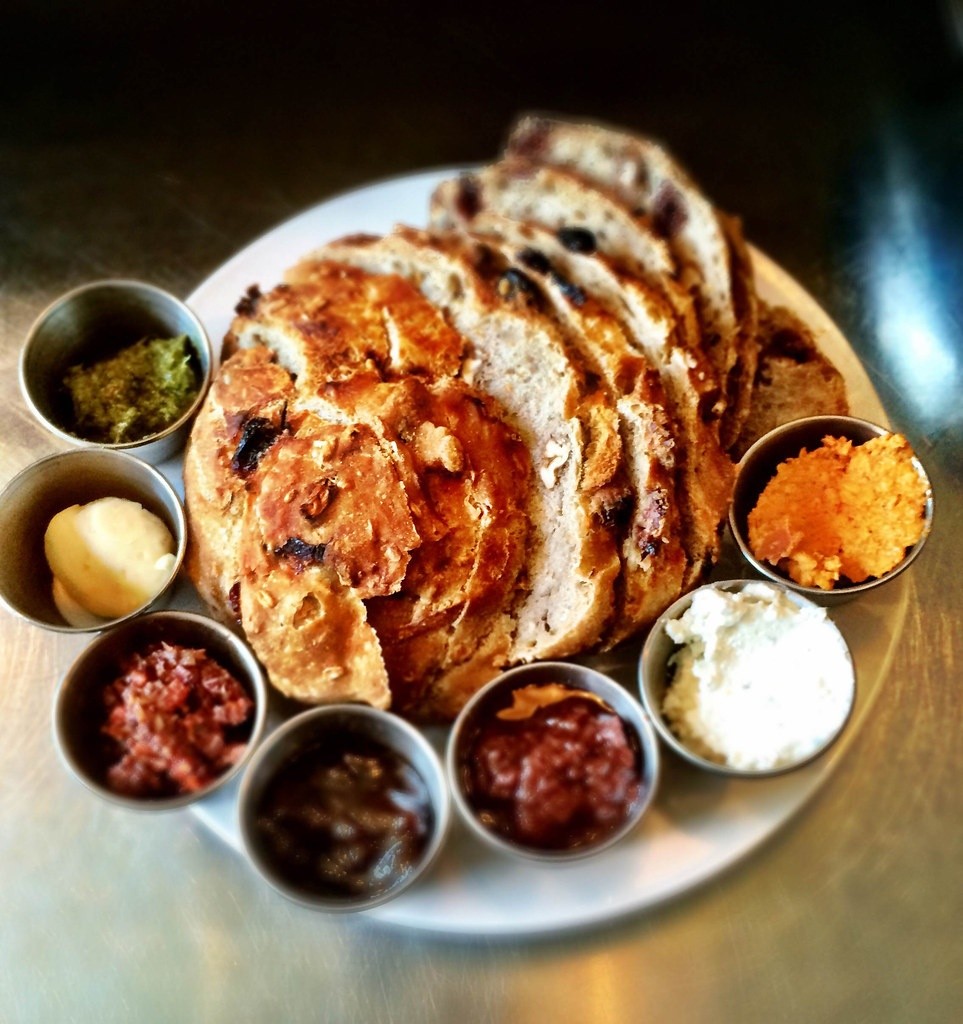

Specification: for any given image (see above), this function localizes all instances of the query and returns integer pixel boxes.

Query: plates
[238,702,452,915]
[114,162,925,942]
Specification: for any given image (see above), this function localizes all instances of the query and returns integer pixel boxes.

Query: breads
[183,113,854,732]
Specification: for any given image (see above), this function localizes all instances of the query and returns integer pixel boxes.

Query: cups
[442,659,660,865]
[0,445,189,636]
[17,277,212,464]
[53,611,266,810]
[724,416,934,605]
[638,577,856,778]
